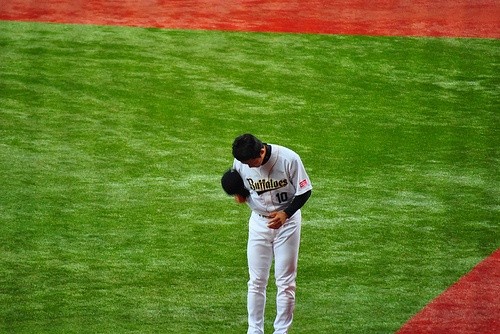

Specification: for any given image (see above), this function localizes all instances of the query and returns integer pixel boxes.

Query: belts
[259,214,275,219]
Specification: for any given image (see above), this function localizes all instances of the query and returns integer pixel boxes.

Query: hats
[221,169,250,198]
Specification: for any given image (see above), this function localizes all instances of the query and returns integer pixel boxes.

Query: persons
[230,133,313,334]
